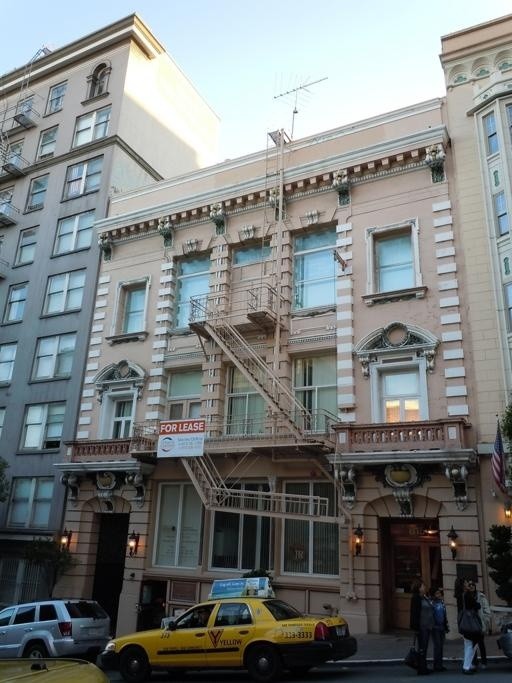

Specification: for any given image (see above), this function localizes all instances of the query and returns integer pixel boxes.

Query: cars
[0,599,110,683]
[497,621,512,658]
[97,597,357,682]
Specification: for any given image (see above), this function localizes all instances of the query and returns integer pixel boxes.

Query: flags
[490,421,506,492]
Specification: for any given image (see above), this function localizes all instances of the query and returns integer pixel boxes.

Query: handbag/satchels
[458,610,482,635]
[404,647,425,669]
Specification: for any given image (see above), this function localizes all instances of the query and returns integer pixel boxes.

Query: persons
[430,588,449,672]
[410,580,436,676]
[454,578,481,675]
[467,579,491,669]
[197,608,211,627]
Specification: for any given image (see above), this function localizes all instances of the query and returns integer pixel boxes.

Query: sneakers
[463,664,487,674]
[417,664,446,675]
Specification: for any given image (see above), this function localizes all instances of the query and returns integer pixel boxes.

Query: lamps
[447,525,460,560]
[128,530,139,557]
[60,528,72,552]
[353,526,364,557]
